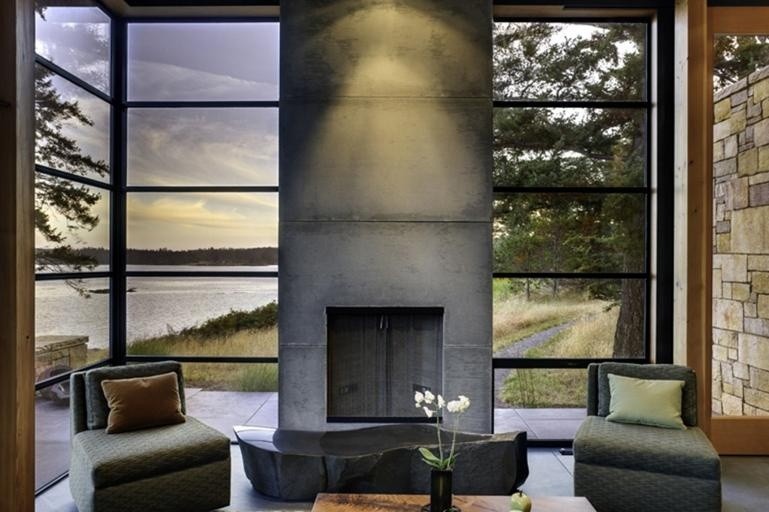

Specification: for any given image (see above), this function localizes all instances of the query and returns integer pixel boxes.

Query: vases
[430,466,452,512]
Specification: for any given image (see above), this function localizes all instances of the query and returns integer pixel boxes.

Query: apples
[508,489,533,512]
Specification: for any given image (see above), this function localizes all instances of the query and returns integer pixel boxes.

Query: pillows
[95,371,189,433]
[604,369,690,431]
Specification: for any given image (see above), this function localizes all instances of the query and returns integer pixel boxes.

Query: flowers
[410,390,472,471]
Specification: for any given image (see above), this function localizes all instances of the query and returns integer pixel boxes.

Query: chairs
[66,364,232,511]
[570,362,723,512]
